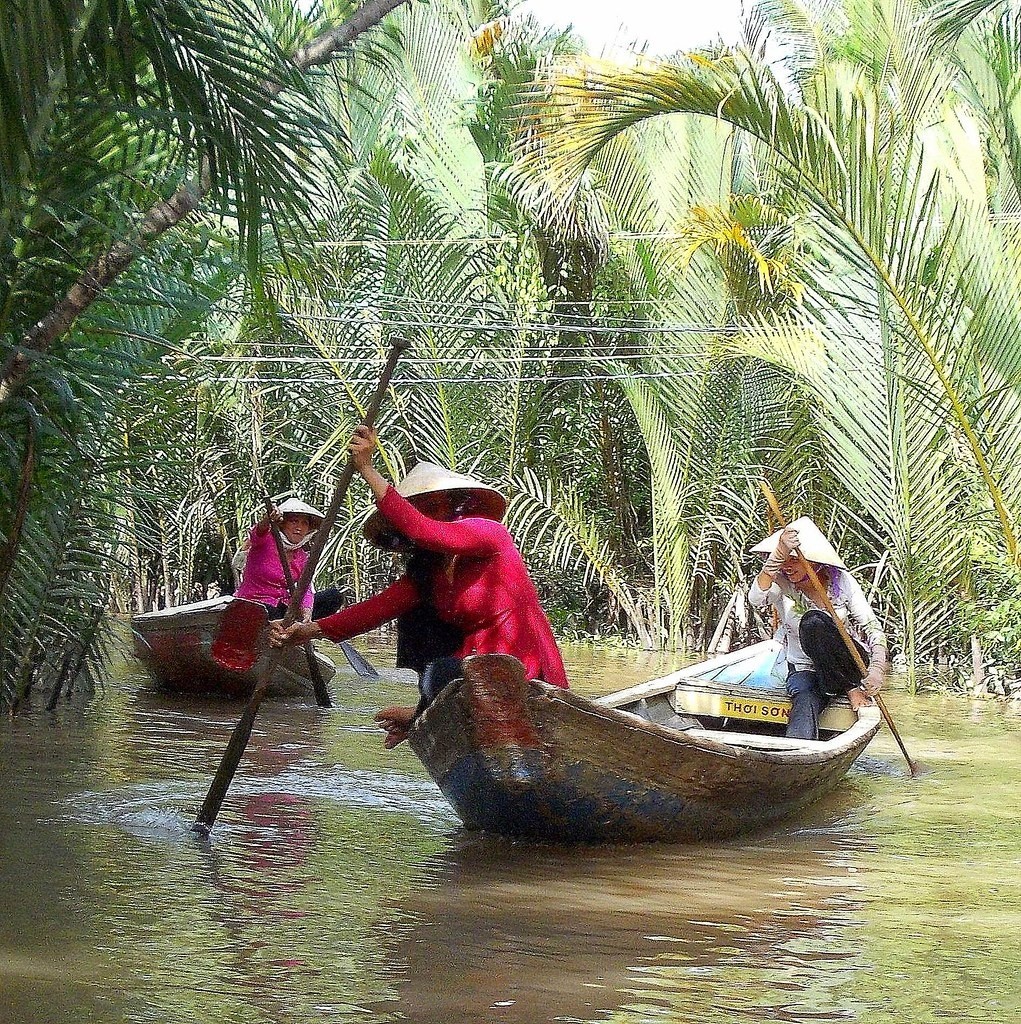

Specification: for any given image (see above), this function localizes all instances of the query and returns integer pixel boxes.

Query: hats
[749,516,850,572]
[363,462,507,553]
[277,498,326,518]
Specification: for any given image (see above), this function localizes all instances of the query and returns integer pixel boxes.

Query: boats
[130,595,336,699]
[407,640,884,846]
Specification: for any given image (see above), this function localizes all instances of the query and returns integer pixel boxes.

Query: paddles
[190,335,413,833]
[757,480,919,778]
[336,639,381,681]
[260,490,329,706]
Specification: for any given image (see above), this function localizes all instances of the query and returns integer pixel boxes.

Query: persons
[236,498,345,622]
[748,516,887,741]
[266,460,570,750]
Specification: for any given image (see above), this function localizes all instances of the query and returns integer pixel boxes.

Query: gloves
[860,645,885,699]
[762,528,801,580]
